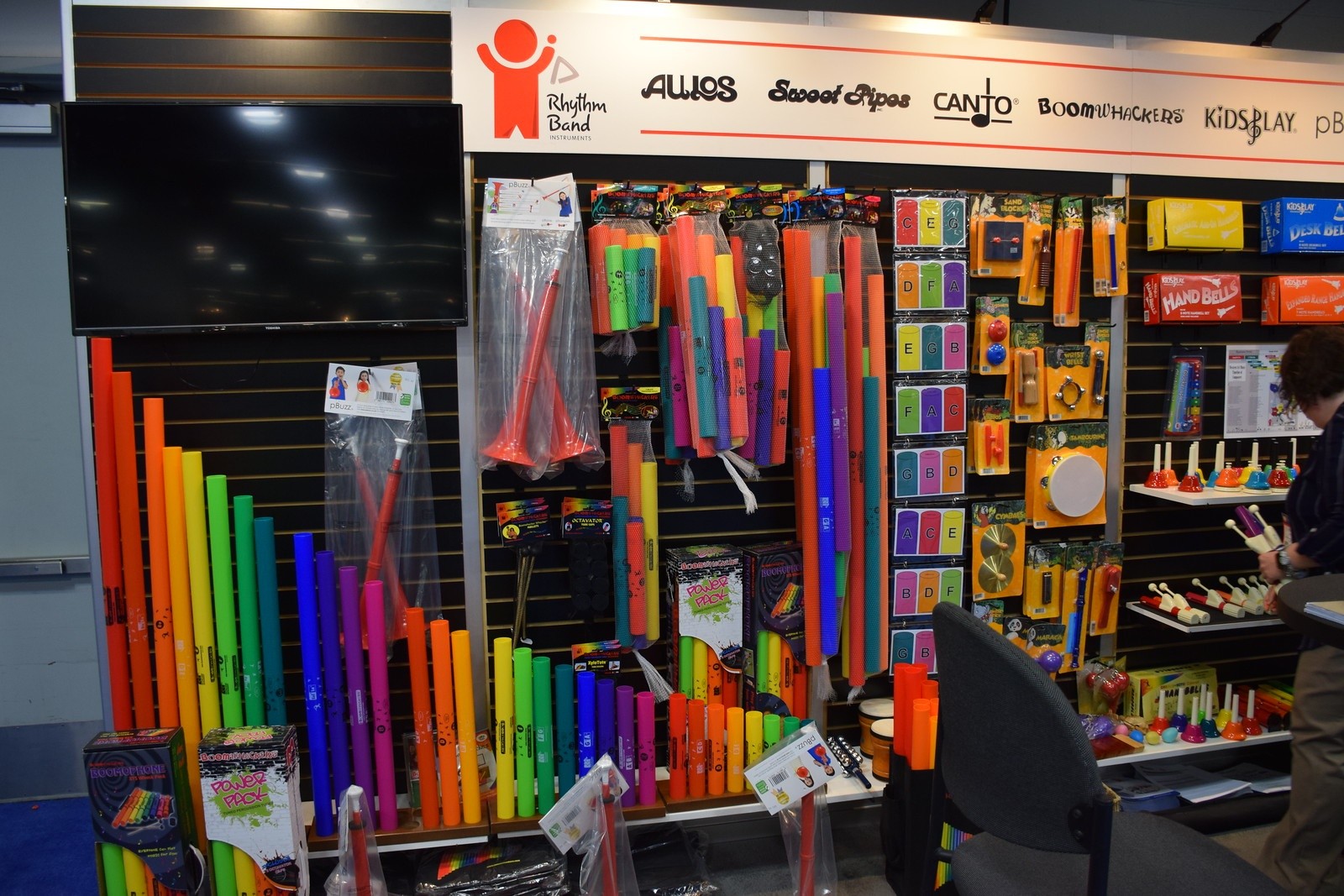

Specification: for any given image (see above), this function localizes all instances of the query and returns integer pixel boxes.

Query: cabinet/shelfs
[1125,456,1297,634]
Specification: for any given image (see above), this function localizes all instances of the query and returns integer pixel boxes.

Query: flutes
[1069,560,1088,668]
[1108,212,1118,291]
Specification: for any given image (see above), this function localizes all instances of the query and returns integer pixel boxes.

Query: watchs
[1276,546,1294,577]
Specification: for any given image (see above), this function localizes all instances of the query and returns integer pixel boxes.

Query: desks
[305,727,1294,896]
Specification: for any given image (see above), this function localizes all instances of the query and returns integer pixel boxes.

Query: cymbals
[1041,455,1063,511]
[978,524,1016,593]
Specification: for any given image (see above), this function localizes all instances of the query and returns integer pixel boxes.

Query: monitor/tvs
[59,101,470,338]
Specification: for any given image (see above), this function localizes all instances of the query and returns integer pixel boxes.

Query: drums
[858,699,894,782]
[1043,452,1106,519]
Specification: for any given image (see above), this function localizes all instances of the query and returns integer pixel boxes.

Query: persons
[600,768,623,797]
[807,743,835,776]
[1253,324,1344,896]
[355,370,371,402]
[541,185,572,217]
[789,756,814,787]
[330,367,348,400]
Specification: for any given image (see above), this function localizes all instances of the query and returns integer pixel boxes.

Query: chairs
[930,601,1298,896]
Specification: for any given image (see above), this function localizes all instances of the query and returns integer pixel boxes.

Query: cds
[978,524,1017,593]
